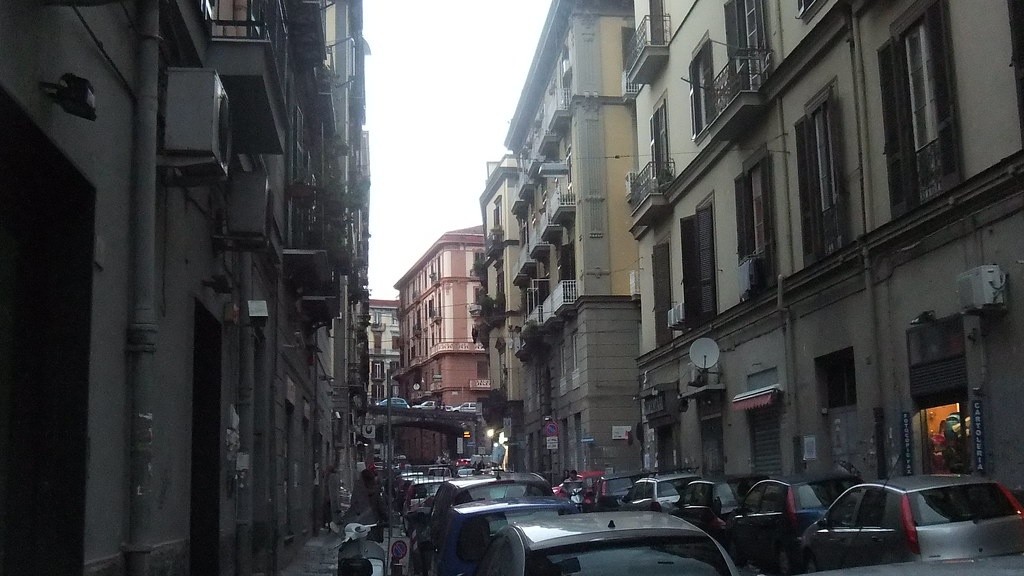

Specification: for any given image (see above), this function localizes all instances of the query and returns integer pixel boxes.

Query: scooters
[558,487,585,515]
[331,522,385,576]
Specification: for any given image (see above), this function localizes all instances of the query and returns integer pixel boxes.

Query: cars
[416,495,581,576]
[371,459,554,576]
[725,475,866,576]
[369,397,410,408]
[459,511,739,576]
[583,473,652,513]
[617,474,702,514]
[669,473,771,541]
[799,474,1024,576]
[452,402,476,413]
[412,401,436,409]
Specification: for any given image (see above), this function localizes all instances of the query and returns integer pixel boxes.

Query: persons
[558,470,578,487]
[345,463,389,545]
